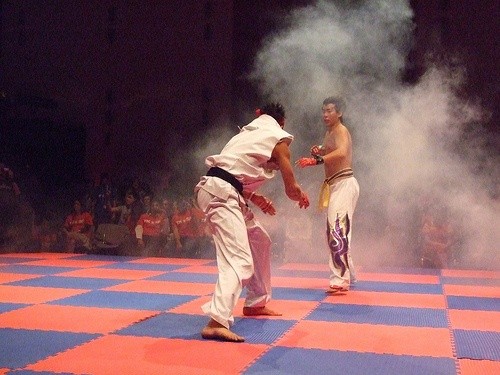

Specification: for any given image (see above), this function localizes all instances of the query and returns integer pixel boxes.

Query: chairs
[86,224,130,255]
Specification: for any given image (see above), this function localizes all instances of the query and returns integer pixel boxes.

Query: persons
[193,102,310,342]
[0,163,500,268]
[294,96,359,296]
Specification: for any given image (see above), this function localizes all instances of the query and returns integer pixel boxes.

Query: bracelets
[314,154,324,164]
